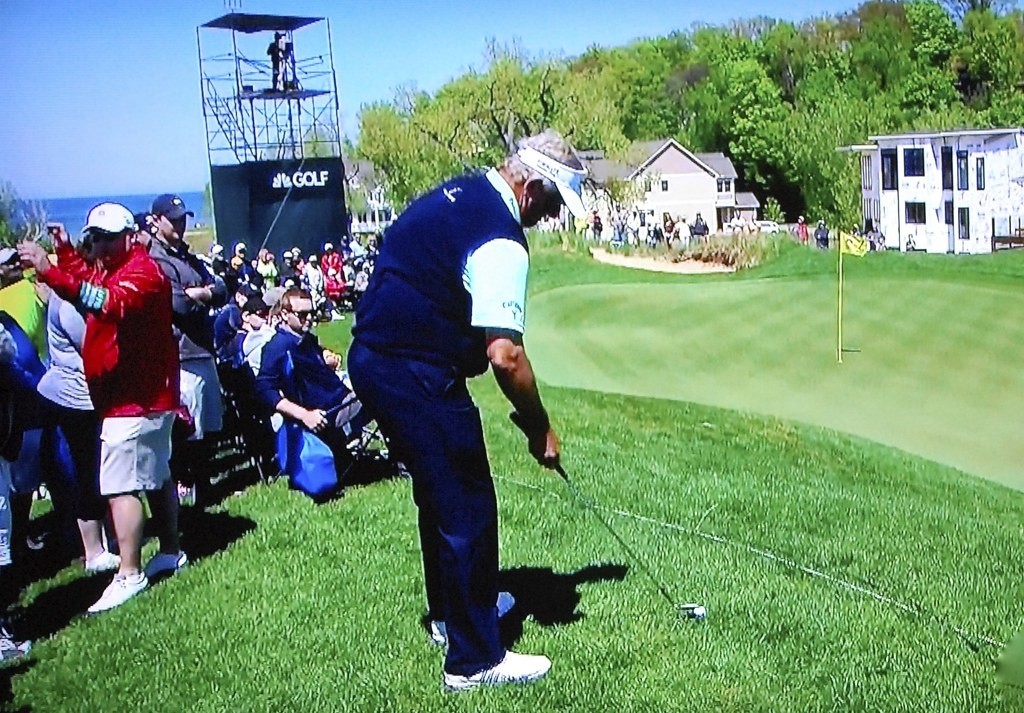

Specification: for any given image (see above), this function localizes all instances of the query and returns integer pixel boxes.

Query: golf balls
[694,606,706,617]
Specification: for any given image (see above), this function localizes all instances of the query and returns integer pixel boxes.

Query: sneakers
[430,592,515,643]
[87,570,152,613]
[143,550,192,585]
[443,650,552,692]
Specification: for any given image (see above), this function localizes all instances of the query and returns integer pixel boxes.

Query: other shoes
[0,638,32,665]
[331,308,346,320]
[177,481,196,506]
[84,551,121,577]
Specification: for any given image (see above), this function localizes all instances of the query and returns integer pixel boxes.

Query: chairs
[213,359,408,492]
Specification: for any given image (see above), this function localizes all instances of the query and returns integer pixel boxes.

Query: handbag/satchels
[290,429,338,498]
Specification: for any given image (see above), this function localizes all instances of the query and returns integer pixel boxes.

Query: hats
[519,147,589,222]
[134,213,158,236]
[210,242,337,287]
[81,202,136,233]
[240,299,272,313]
[151,193,195,220]
[0,247,19,264]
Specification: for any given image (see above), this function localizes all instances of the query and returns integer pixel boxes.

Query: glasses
[90,231,118,244]
[290,309,318,319]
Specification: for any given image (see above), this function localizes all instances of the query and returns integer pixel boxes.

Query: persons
[268,32,285,88]
[347,128,589,690]
[0,194,383,673]
[591,199,860,261]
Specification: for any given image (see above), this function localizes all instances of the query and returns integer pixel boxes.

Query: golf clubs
[323,395,358,417]
[509,410,699,617]
